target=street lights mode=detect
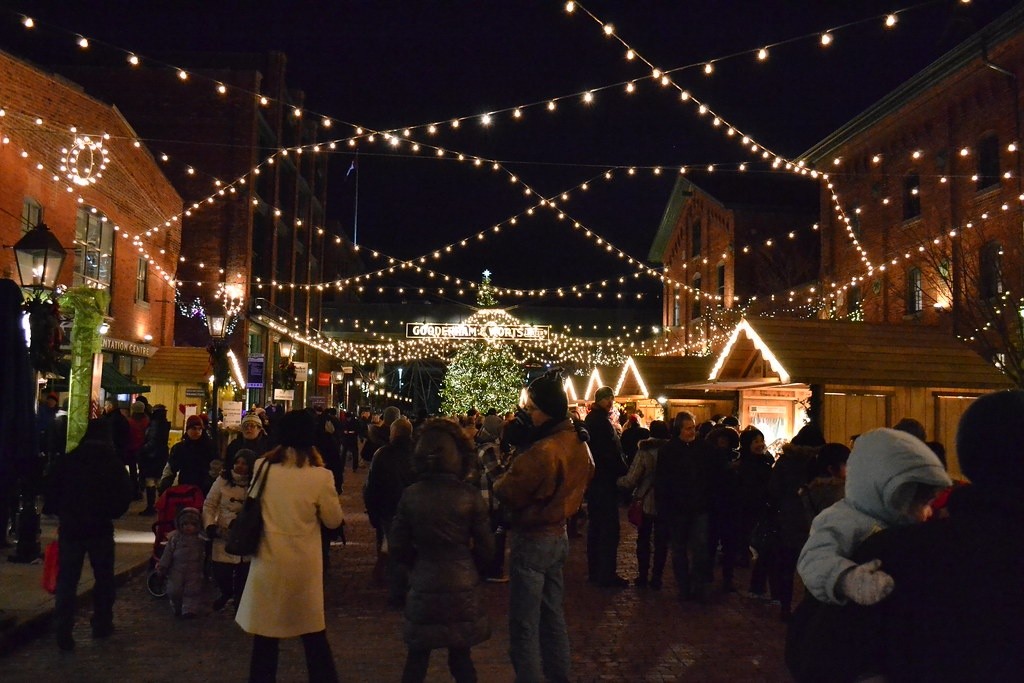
[335,364,345,411]
[279,333,296,413]
[12,224,68,559]
[204,300,228,457]
[368,379,376,411]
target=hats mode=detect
[529,368,566,393]
[815,443,852,477]
[47,391,59,402]
[233,449,257,471]
[179,513,202,530]
[131,402,145,413]
[186,414,210,431]
[629,415,637,423]
[530,379,568,420]
[241,414,262,428]
[956,390,1024,487]
[595,386,615,402]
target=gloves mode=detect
[576,425,592,442]
[839,559,894,606]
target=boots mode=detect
[566,515,583,537]
[137,483,158,517]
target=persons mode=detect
[225,412,270,469]
[774,424,825,491]
[38,391,68,465]
[788,390,1024,683]
[203,449,256,613]
[156,507,206,615]
[796,427,951,612]
[490,369,594,683]
[34,418,131,649]
[157,416,218,495]
[241,405,413,564]
[388,417,495,683]
[453,409,741,591]
[102,394,171,516]
[926,440,972,517]
[231,410,347,683]
[585,387,630,587]
[496,367,589,537]
[795,443,852,563]
[734,426,783,605]
[895,417,927,443]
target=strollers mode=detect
[146,483,211,597]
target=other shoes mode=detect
[634,573,649,587]
[374,551,389,573]
[485,574,509,583]
[587,572,631,587]
[649,567,664,589]
[7,554,38,563]
[92,622,114,636]
[55,617,75,651]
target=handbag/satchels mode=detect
[629,495,645,525]
[225,457,272,556]
